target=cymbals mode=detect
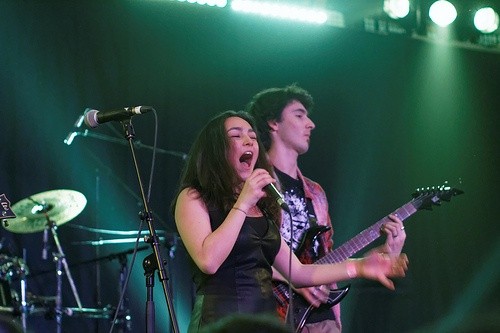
[2,189,87,234]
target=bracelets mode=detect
[347,258,357,277]
[232,207,247,215]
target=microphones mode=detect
[263,184,289,214]
[84,105,154,129]
[63,114,85,145]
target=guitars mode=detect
[273,186,464,332]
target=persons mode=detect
[247,87,406,333]
[171,111,409,333]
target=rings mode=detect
[313,289,319,296]
[315,285,323,290]
[394,235,397,237]
[401,226,404,230]
